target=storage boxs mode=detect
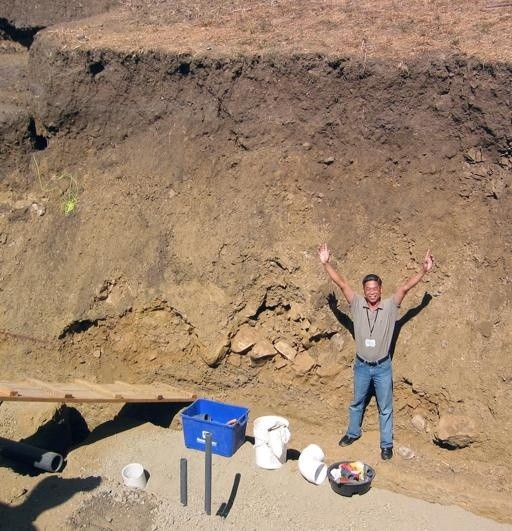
[179,398,250,457]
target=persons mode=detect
[315,242,435,460]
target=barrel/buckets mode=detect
[253,415,290,469]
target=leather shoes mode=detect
[380,446,393,460]
[338,436,360,448]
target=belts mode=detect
[356,354,389,368]
